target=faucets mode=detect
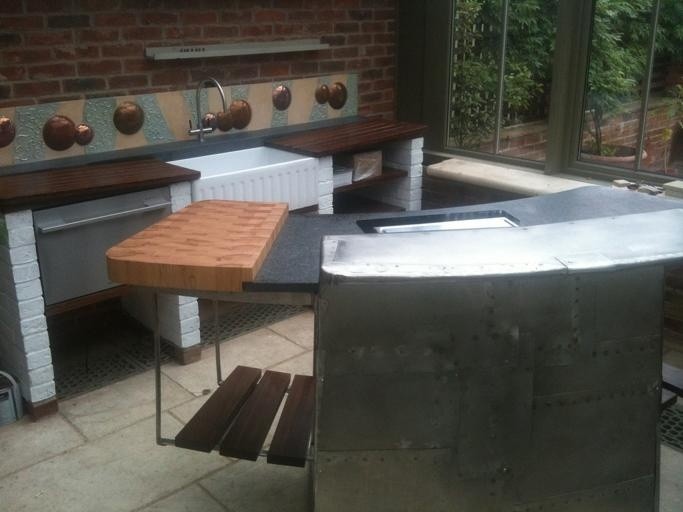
[187,76,227,142]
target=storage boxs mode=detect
[332,149,383,188]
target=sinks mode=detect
[354,210,521,235]
[165,144,318,213]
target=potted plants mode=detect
[577,0,647,170]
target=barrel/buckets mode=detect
[0,372,23,423]
